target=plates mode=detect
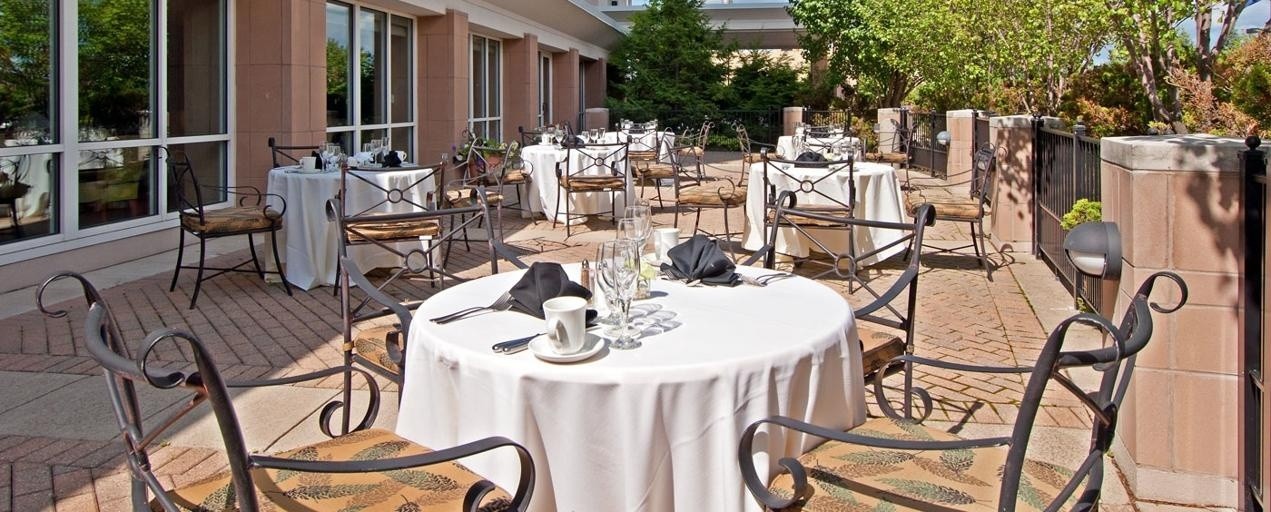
[395,151,406,161]
[297,169,320,174]
[643,252,673,266]
[528,333,604,363]
[539,143,550,146]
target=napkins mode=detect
[659,236,741,287]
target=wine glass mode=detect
[791,122,858,160]
[614,118,659,132]
[319,137,389,173]
[606,303,680,341]
[595,205,652,349]
[547,126,604,148]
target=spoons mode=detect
[741,273,794,287]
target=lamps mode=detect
[1065,220,1122,281]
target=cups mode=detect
[299,156,316,171]
[654,229,679,261]
[542,135,548,144]
[542,296,587,355]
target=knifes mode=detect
[492,323,598,354]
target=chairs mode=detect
[746,126,915,294]
[39,269,536,511]
[663,139,749,244]
[265,138,514,306]
[462,118,714,237]
[903,144,1000,282]
[168,150,294,307]
[739,187,939,427]
[324,187,501,436]
[738,273,1189,510]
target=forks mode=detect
[428,291,514,324]
